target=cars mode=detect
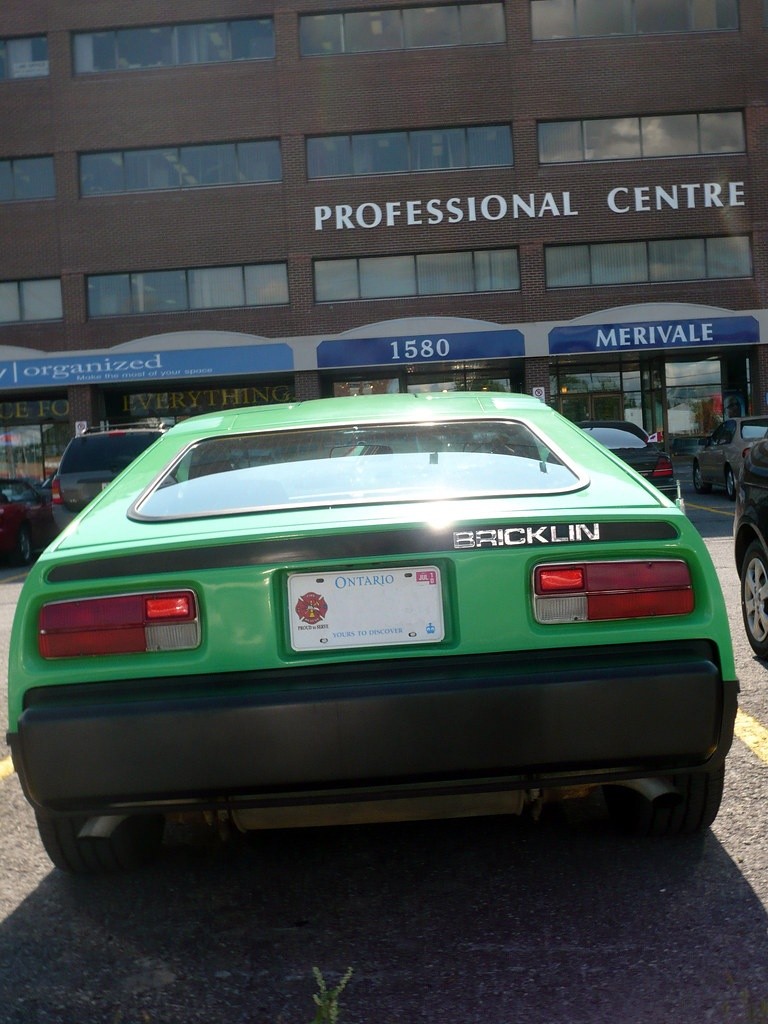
[693,415,768,502]
[733,429,768,663]
[0,479,56,569]
[573,419,677,503]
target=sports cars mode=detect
[6,392,742,909]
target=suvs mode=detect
[50,421,171,533]
